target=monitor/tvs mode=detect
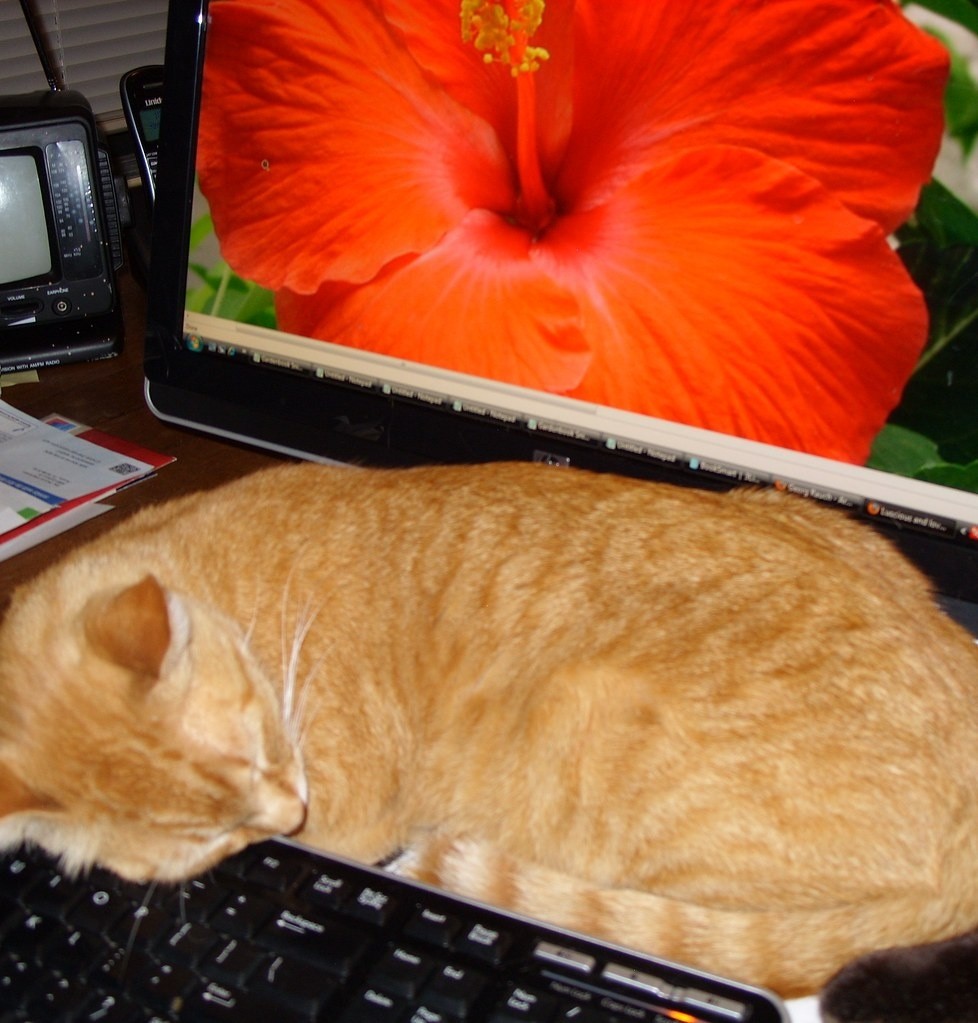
[140,0,978,641]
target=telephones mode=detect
[120,63,164,280]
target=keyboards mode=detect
[1,838,789,1023]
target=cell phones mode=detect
[117,65,166,202]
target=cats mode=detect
[0,462,978,1003]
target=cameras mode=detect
[1,89,122,374]
